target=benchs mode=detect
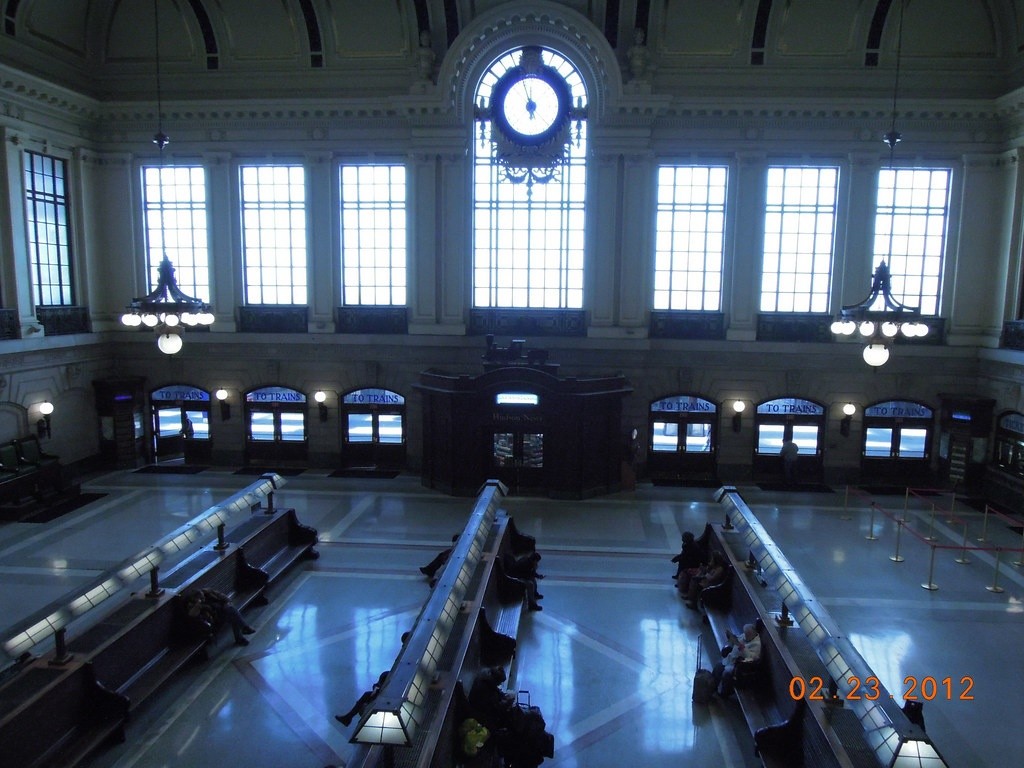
[695,522,854,768]
[0,508,321,768]
[340,483,536,768]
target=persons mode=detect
[682,550,728,608]
[671,532,706,588]
[420,535,459,578]
[781,437,799,487]
[503,552,542,611]
[193,587,256,646]
[711,624,762,699]
[517,553,544,599]
[335,671,390,727]
[468,665,506,708]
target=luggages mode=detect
[692,633,716,704]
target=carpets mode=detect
[1007,527,1024,535]
[859,485,915,496]
[18,493,110,523]
[327,470,401,479]
[651,478,724,488]
[955,497,997,514]
[232,467,307,477]
[132,466,211,474]
[756,483,835,493]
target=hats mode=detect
[491,665,507,682]
[682,532,694,541]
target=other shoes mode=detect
[241,627,256,635]
[237,637,250,645]
[675,584,679,588]
[528,602,542,612]
[672,574,678,578]
[419,566,435,578]
[685,601,698,610]
[535,592,543,599]
[335,715,352,727]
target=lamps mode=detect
[348,479,509,747]
[831,0,929,366]
[122,0,215,354]
[314,389,327,423]
[0,473,287,659]
[37,400,55,439]
[715,486,950,768]
[215,386,231,421]
[840,401,856,438]
[732,399,746,433]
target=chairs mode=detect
[0,435,63,503]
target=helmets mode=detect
[722,645,732,658]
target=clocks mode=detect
[489,50,573,168]
